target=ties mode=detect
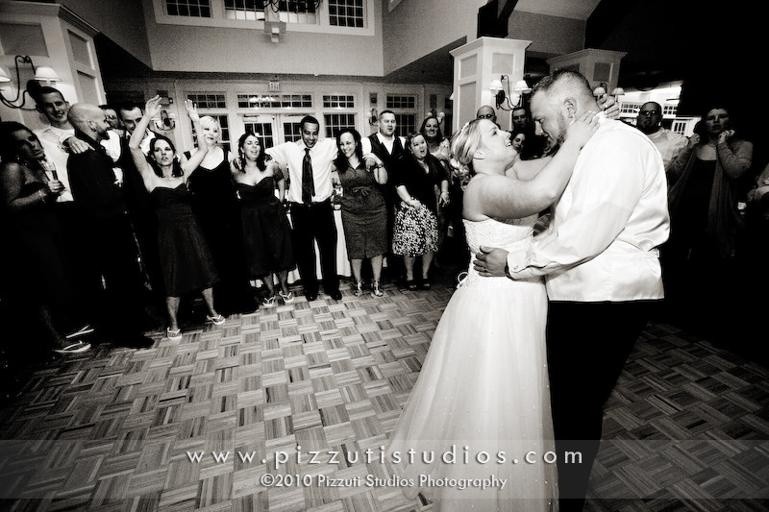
[302,147,315,207]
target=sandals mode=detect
[369,278,382,296]
[352,279,363,297]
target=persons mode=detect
[0,67,769,512]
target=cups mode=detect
[41,161,61,194]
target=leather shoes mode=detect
[406,277,431,291]
[305,290,341,302]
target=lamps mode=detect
[592,82,624,101]
[491,74,531,110]
[152,112,175,131]
[0,54,58,111]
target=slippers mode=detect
[262,294,279,308]
[207,312,226,327]
[167,325,182,340]
[278,289,295,303]
[66,325,94,340]
[52,340,91,354]
